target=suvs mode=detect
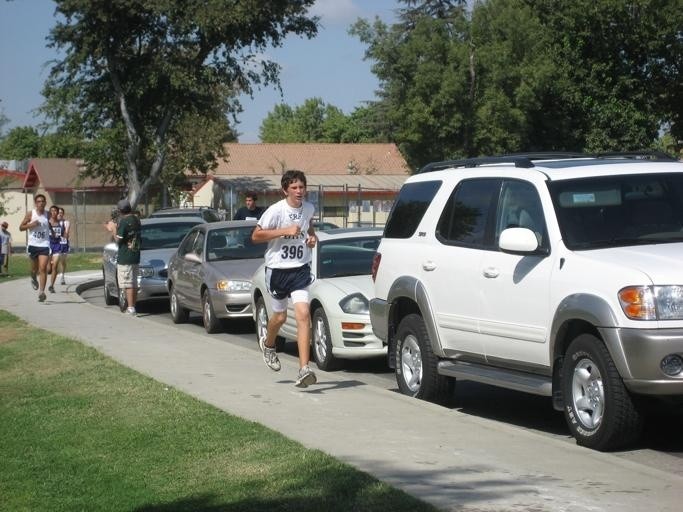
[369,150,682,452]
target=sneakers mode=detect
[31,276,65,303]
[259,336,282,372]
[122,309,137,318]
[294,366,317,389]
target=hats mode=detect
[117,199,131,210]
[0,221,9,226]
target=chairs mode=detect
[624,190,673,237]
[210,235,226,252]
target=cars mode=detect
[168,218,269,335]
[148,204,220,224]
[249,225,392,370]
[101,217,209,312]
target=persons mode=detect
[250,170,317,388]
[233,193,265,221]
[20,194,52,302]
[104,199,141,317]
[0,221,11,274]
[47,205,64,293]
[56,208,71,285]
